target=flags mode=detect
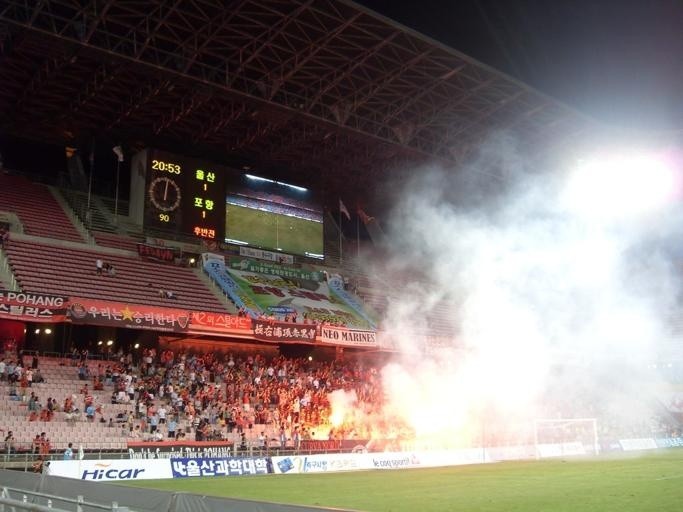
[356,204,373,225]
[339,199,351,220]
[112,145,124,162]
[63,131,81,164]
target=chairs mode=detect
[0,351,163,449]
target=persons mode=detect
[106,262,115,277]
[37,432,48,458]
[166,288,177,299]
[61,346,385,451]
[95,256,103,276]
[238,307,346,328]
[158,286,164,299]
[0,339,60,422]
[31,457,42,473]
[45,437,51,453]
[63,441,73,461]
[4,430,16,458]
[30,434,40,455]
